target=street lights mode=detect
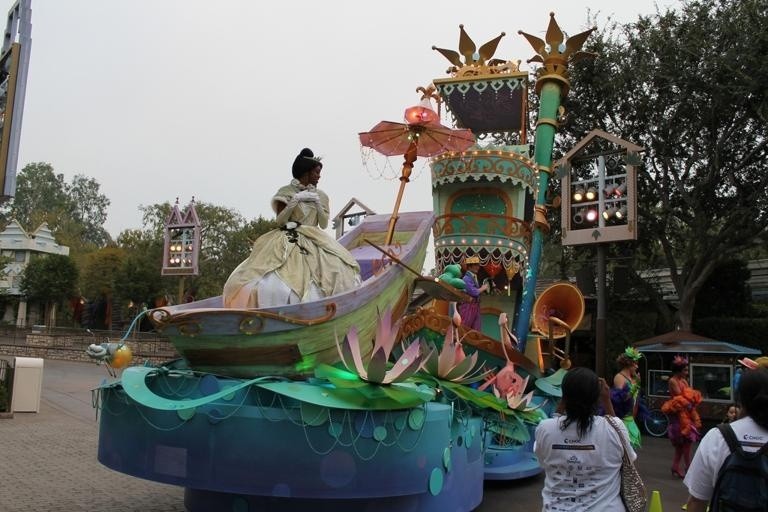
[160,197,202,308]
[552,129,647,380]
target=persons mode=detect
[661,356,768,512]
[223,148,362,309]
[533,366,638,512]
[612,350,643,451]
[460,256,487,332]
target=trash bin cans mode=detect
[11,356,44,414]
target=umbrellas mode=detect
[357,118,478,246]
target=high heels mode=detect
[671,467,685,479]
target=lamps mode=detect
[573,177,628,224]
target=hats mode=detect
[464,257,480,264]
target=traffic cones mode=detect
[648,490,664,512]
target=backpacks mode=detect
[709,424,768,512]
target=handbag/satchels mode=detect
[605,414,647,512]
